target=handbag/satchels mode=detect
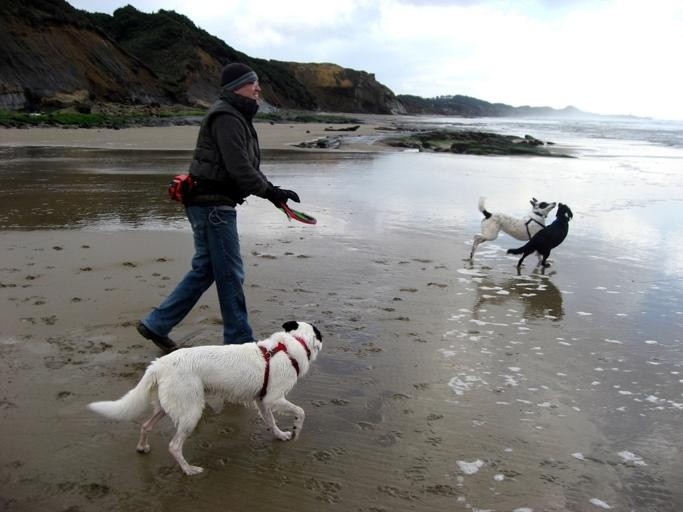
[169,173,197,203]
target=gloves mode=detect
[264,186,289,208]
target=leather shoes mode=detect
[137,322,178,353]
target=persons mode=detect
[136,61,301,353]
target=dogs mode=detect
[85,320,325,477]
[506,202,573,268]
[470,196,557,259]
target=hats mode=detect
[220,62,258,95]
